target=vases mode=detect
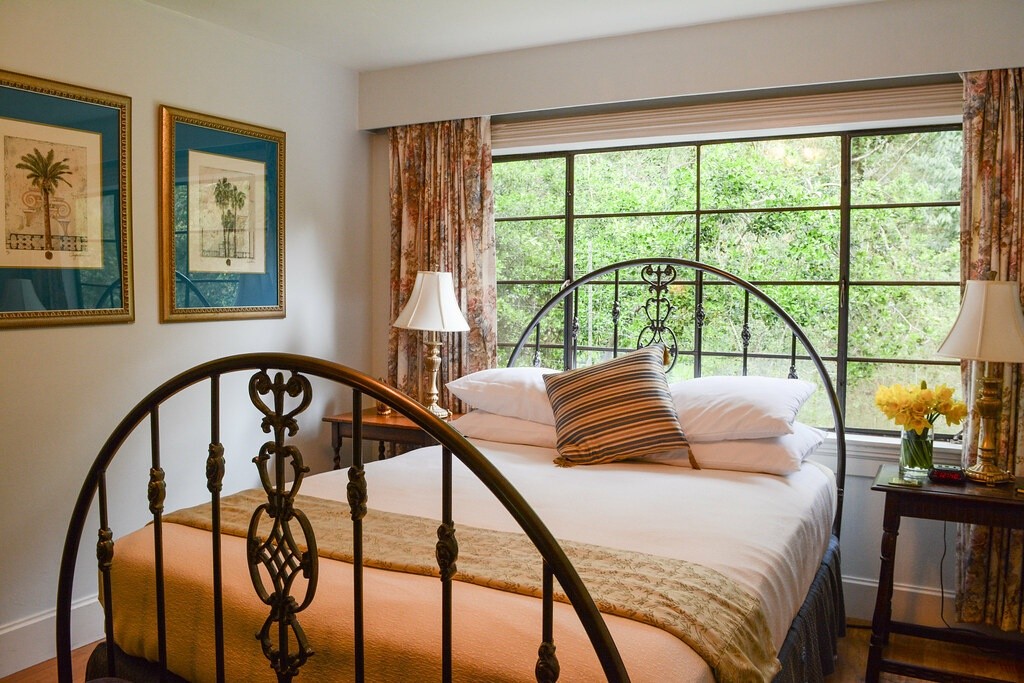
[900,427,936,478]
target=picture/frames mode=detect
[1,69,137,327]
[156,104,285,325]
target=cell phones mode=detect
[887,475,923,487]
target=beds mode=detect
[51,256,849,683]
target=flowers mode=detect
[876,378,970,469]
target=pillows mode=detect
[539,342,690,470]
[668,374,820,444]
[448,409,558,449]
[445,366,562,427]
[690,418,830,477]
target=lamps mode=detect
[932,279,1024,485]
[392,269,472,418]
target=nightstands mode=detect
[861,458,1024,683]
[322,401,441,475]
